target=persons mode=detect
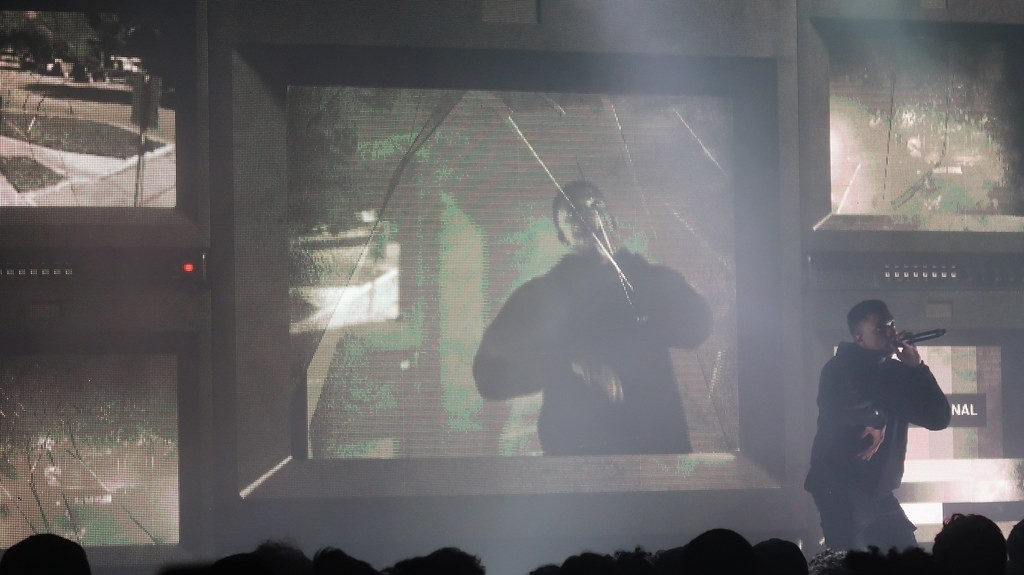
[470,178,717,457]
[803,298,952,552]
[2,513,1024,574]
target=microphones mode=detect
[894,329,946,347]
